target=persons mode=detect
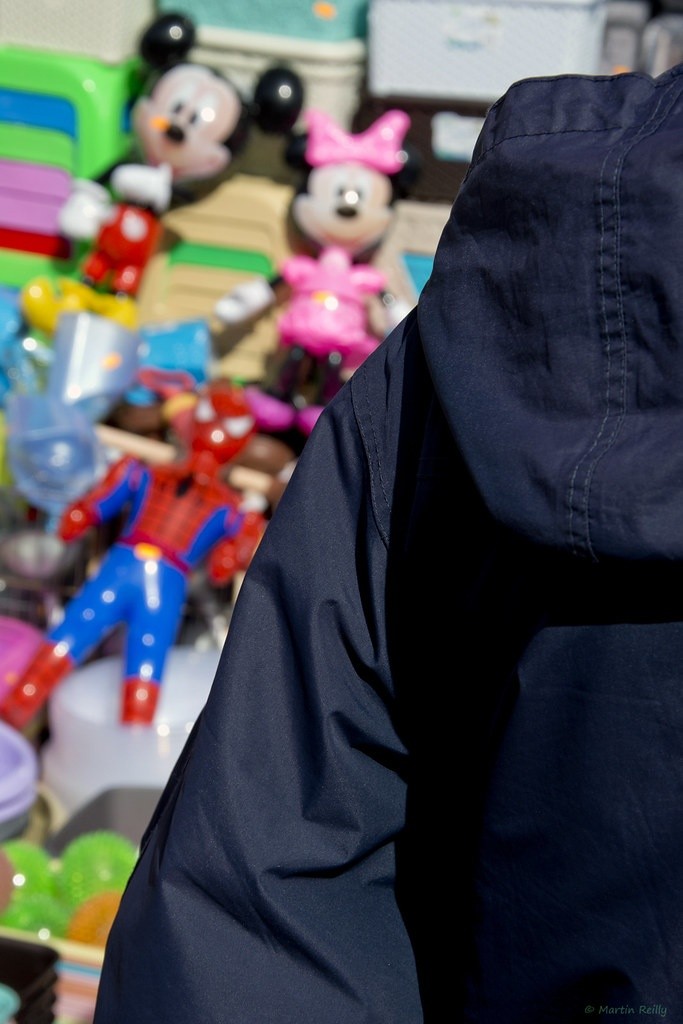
[83,0,682,1024]
[2,381,269,741]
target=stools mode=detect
[1,39,451,403]
